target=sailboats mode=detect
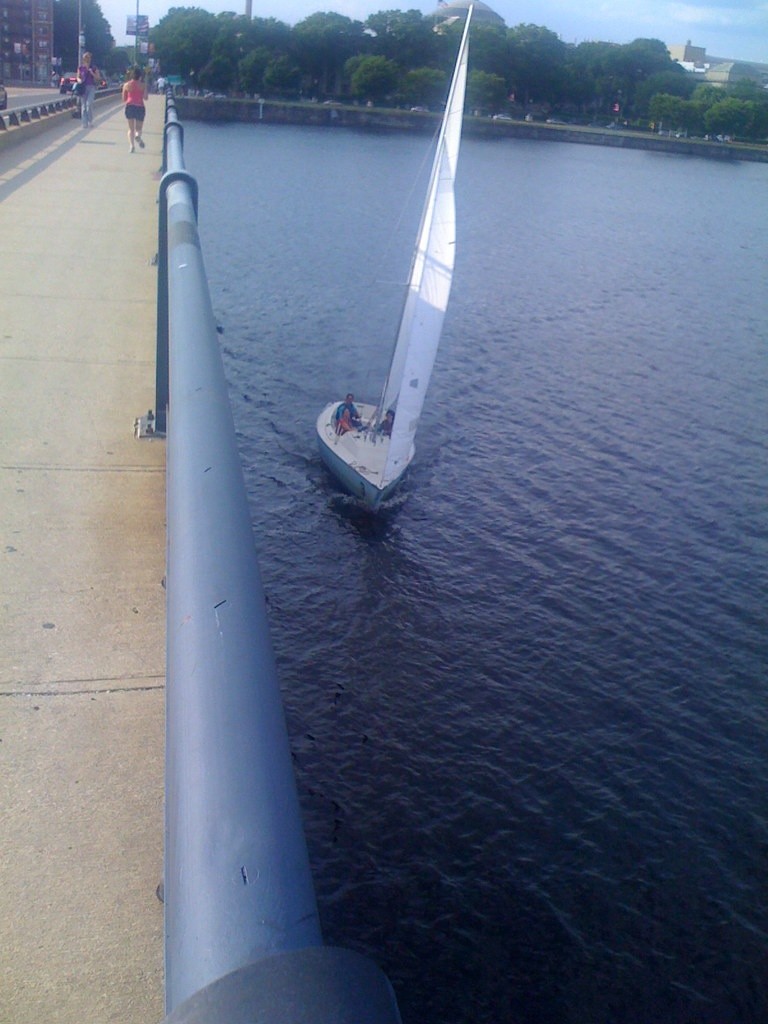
[314,3,475,508]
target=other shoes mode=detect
[135,136,144,148]
[130,147,135,153]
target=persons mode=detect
[157,75,166,95]
[52,69,60,88]
[76,51,100,129]
[377,410,395,436]
[122,68,148,153]
[334,394,368,436]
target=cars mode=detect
[411,106,430,112]
[59,72,78,94]
[95,68,110,90]
[323,99,343,108]
[205,92,228,100]
[493,114,512,121]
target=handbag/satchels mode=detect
[72,81,85,95]
